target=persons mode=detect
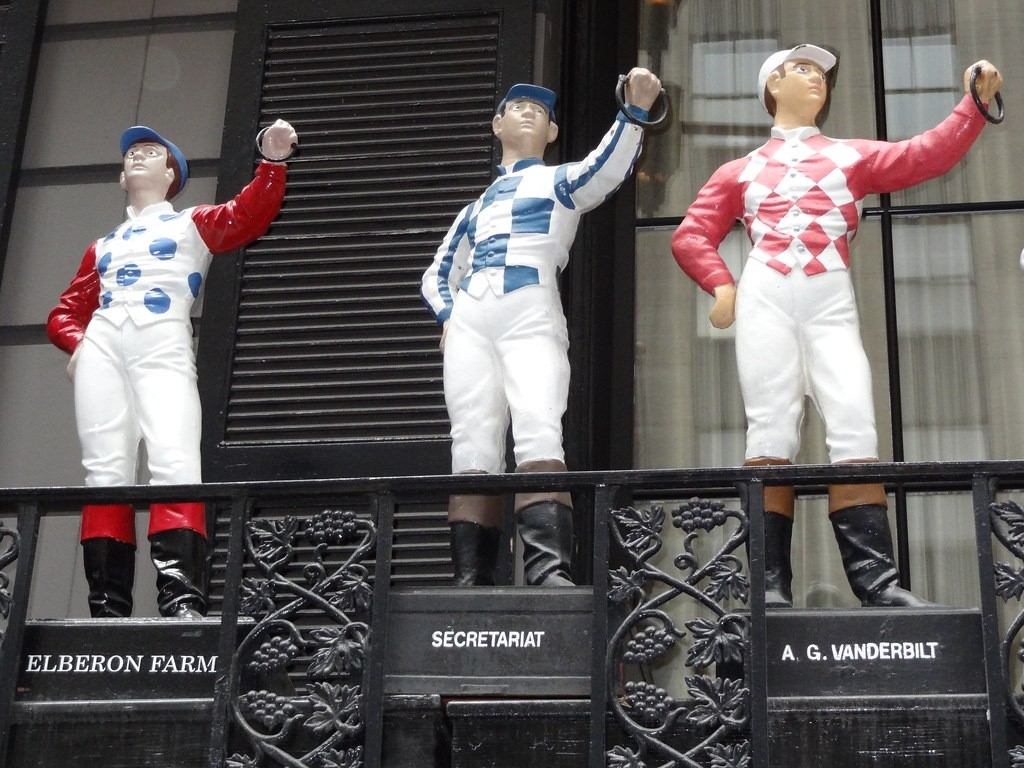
[670,40,1006,611]
[44,117,301,618]
[421,66,677,590]
